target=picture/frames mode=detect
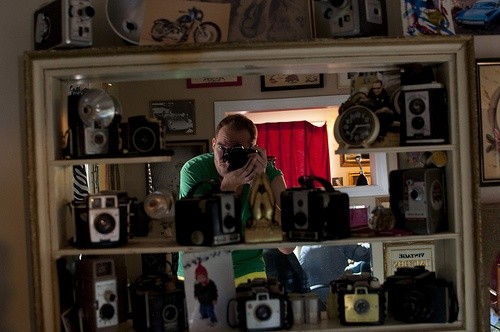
[476,58,500,187]
[349,172,372,186]
[340,154,371,167]
[383,242,434,281]
[149,98,198,136]
[145,139,209,197]
[186,75,242,89]
[260,73,325,92]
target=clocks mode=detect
[334,92,380,149]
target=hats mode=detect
[195,265,207,275]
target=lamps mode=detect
[355,156,369,185]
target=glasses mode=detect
[372,87,381,90]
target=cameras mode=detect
[33,0,458,332]
[223,144,259,171]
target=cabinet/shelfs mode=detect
[21,32,491,332]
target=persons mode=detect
[178,114,373,311]
[193,264,219,325]
[359,79,396,138]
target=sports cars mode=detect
[455,0,500,27]
